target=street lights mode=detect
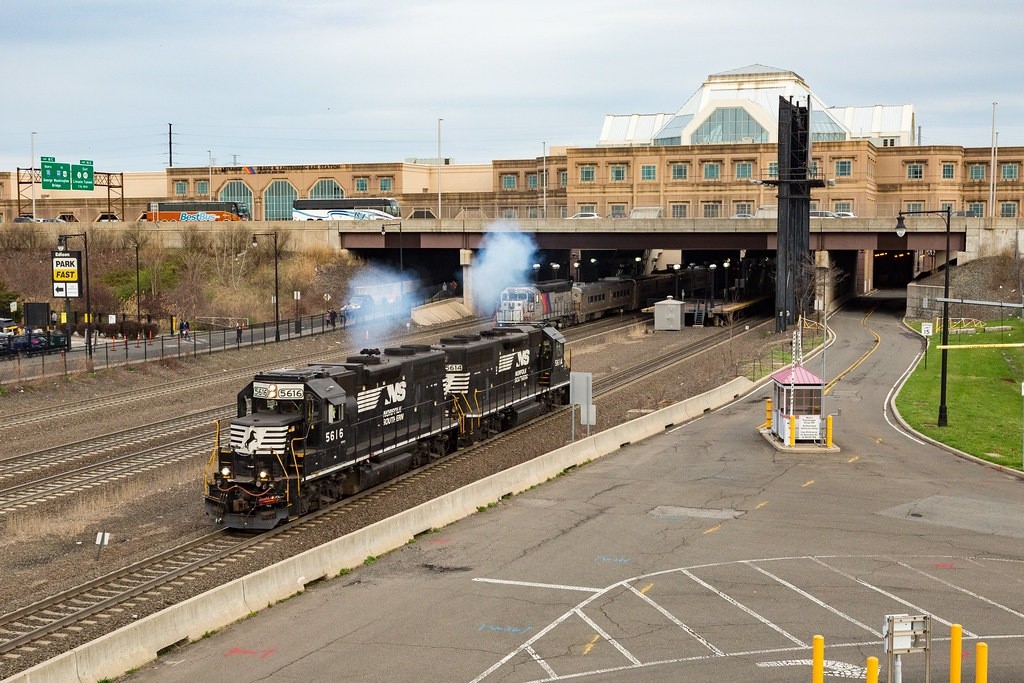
[207,150,211,201]
[382,222,404,309]
[253,232,280,342]
[989,101,1000,217]
[895,207,951,427]
[673,262,730,308]
[32,131,37,223]
[439,118,444,219]
[542,141,546,218]
[57,232,92,359]
[533,257,641,282]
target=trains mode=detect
[204,324,572,530]
[495,266,740,329]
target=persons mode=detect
[442,281,448,297]
[236,327,242,343]
[51,310,58,325]
[179,319,190,340]
[326,308,337,324]
[450,280,457,297]
[544,345,552,369]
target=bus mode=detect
[292,198,401,221]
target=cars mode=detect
[14,217,64,223]
[147,201,250,222]
[0,318,48,356]
[346,296,377,318]
[733,211,856,218]
[564,213,602,219]
